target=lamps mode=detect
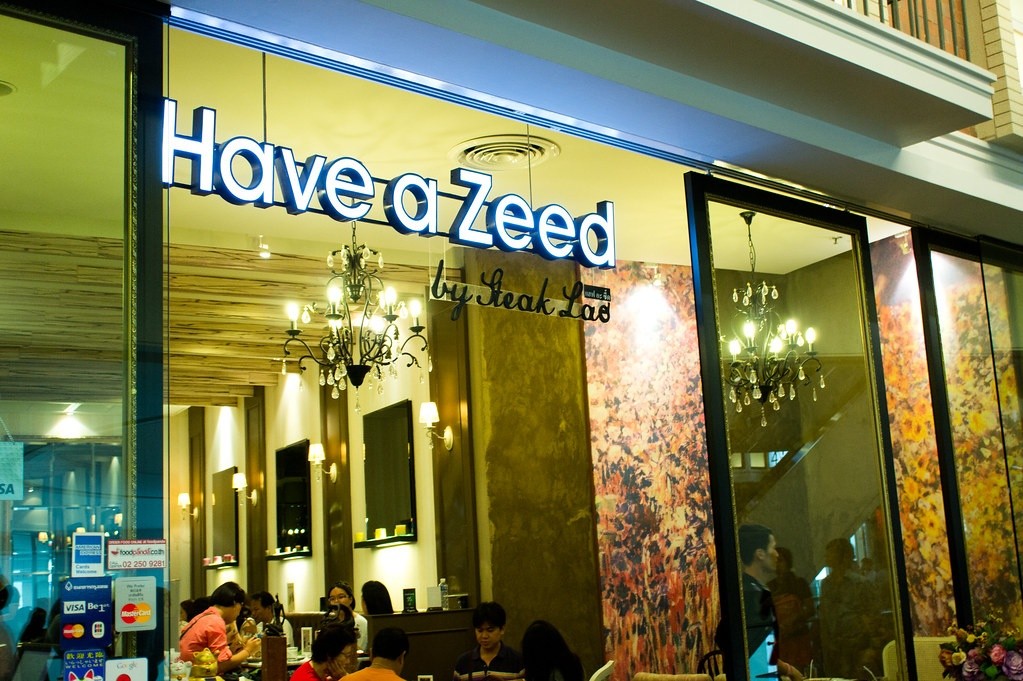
[177,493,199,520]
[419,402,454,451]
[307,443,338,484]
[722,211,826,427]
[282,194,434,416]
[231,473,257,507]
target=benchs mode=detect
[279,611,335,652]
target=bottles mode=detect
[438,578,449,610]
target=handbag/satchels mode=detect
[773,593,804,635]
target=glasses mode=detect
[341,651,352,659]
[328,594,349,602]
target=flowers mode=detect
[936,609,1023,681]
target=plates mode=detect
[246,658,262,663]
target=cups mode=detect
[287,647,298,660]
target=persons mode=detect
[178,581,587,681]
[738,522,890,681]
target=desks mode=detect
[238,651,370,681]
